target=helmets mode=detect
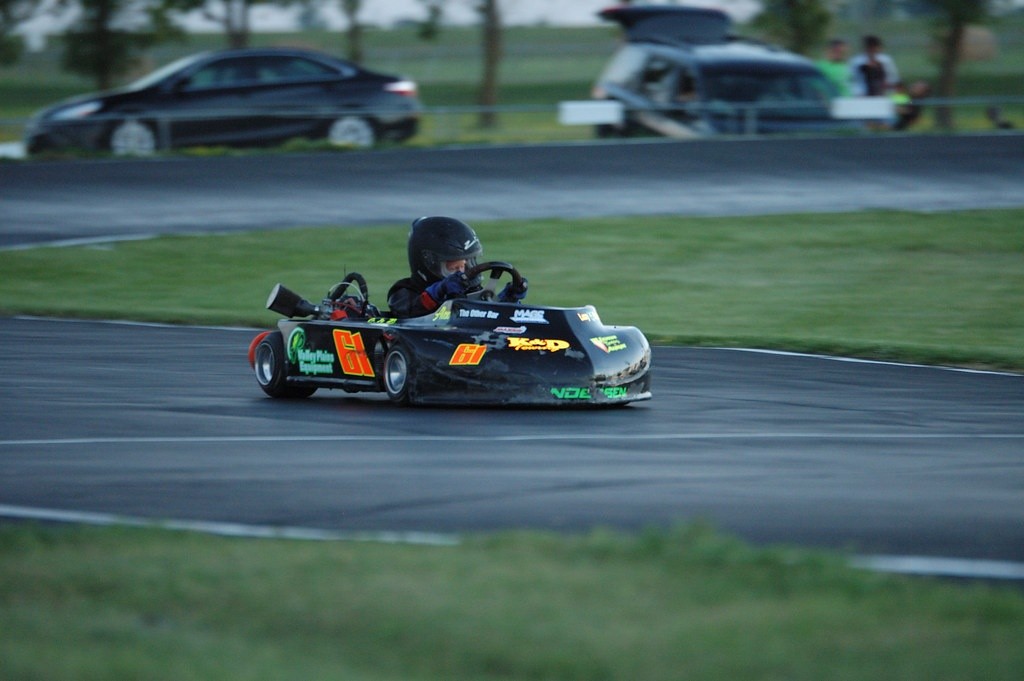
[408,217,483,297]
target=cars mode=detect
[26,46,420,156]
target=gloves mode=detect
[497,277,528,304]
[425,271,482,305]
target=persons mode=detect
[820,38,852,96]
[849,35,900,97]
[387,215,529,318]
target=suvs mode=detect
[591,5,897,139]
